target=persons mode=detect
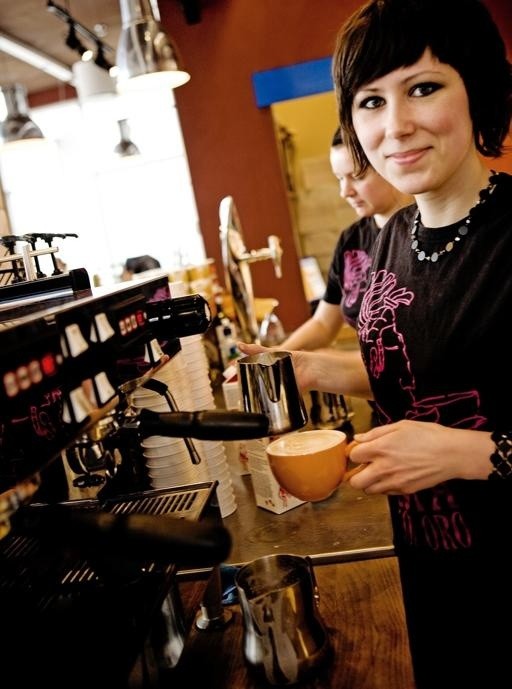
[235,1,512,689]
[272,126,415,352]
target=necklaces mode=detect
[410,170,499,263]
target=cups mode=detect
[266,428,370,504]
[127,332,239,520]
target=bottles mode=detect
[221,329,247,368]
[236,553,336,689]
[308,389,357,430]
[235,350,309,436]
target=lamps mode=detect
[65,19,116,78]
[0,84,44,143]
[116,0,192,91]
[114,118,138,156]
[71,60,117,111]
